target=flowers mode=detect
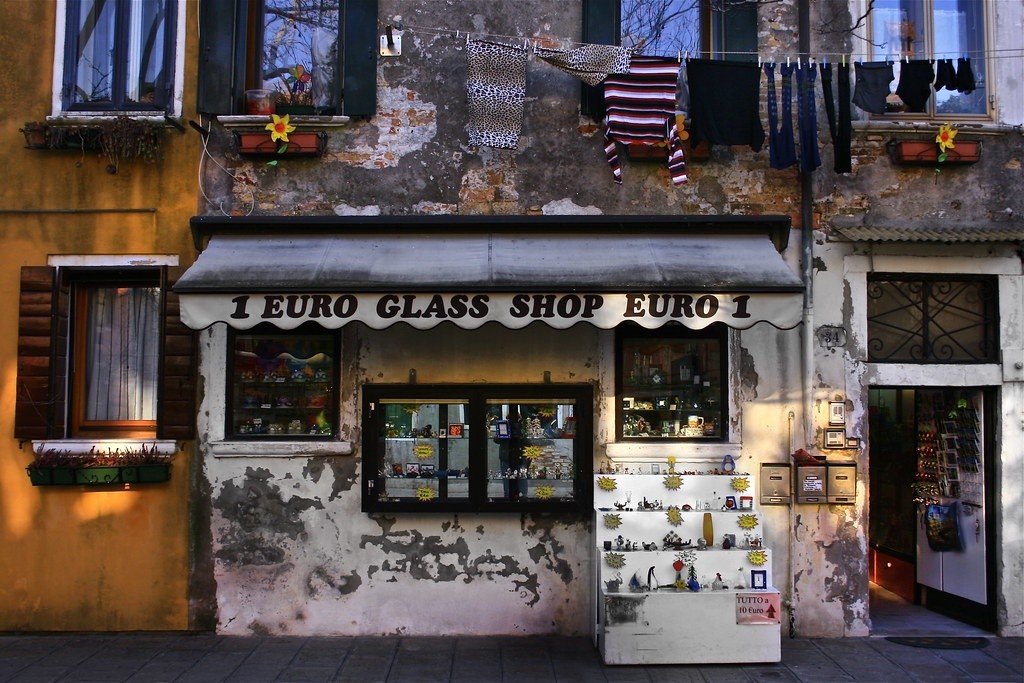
[264,113,297,176]
[287,63,311,94]
[934,122,958,185]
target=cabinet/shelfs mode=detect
[593,474,782,664]
[385,436,574,480]
[624,383,705,412]
[235,380,330,414]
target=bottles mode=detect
[629,353,653,385]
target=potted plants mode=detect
[19,121,101,147]
[27,441,172,487]
[273,84,315,115]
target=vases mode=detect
[239,132,319,152]
[896,141,978,161]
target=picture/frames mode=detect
[449,422,464,438]
[496,420,511,438]
[421,464,435,470]
[941,421,960,481]
[406,463,420,476]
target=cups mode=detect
[703,513,713,546]
[688,415,704,429]
[245,89,275,114]
